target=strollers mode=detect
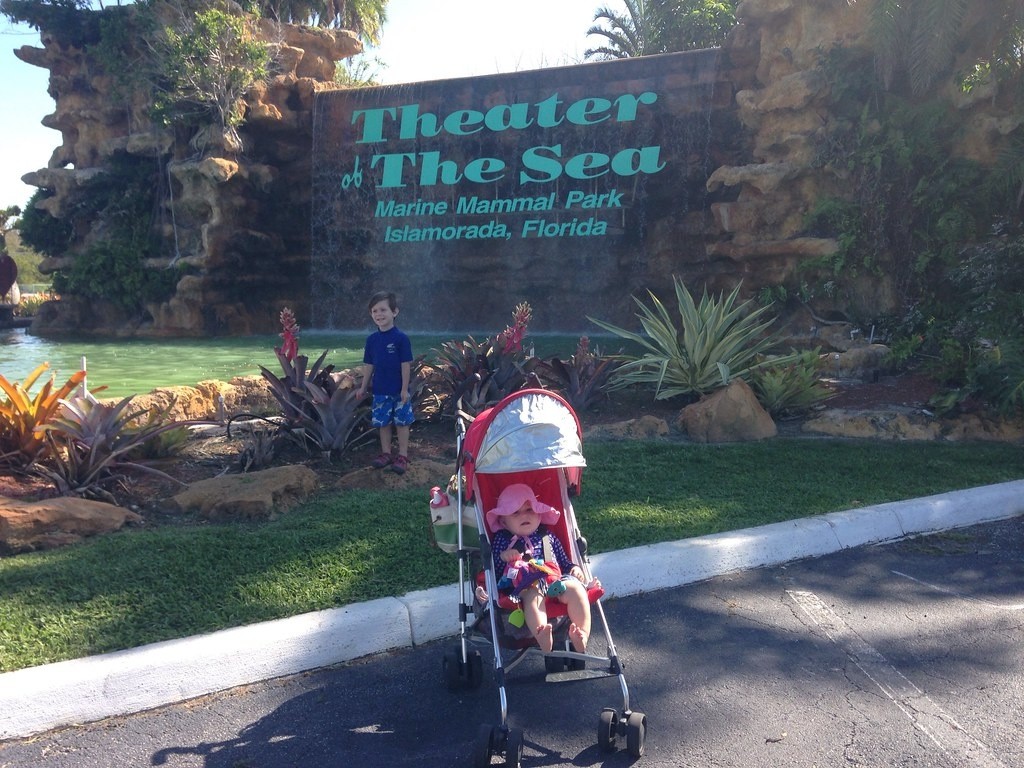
[427,387,651,768]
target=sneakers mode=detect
[392,454,411,473]
[372,453,392,467]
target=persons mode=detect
[487,484,590,653]
[356,292,416,473]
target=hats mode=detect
[486,484,561,533]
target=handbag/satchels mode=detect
[430,441,481,553]
